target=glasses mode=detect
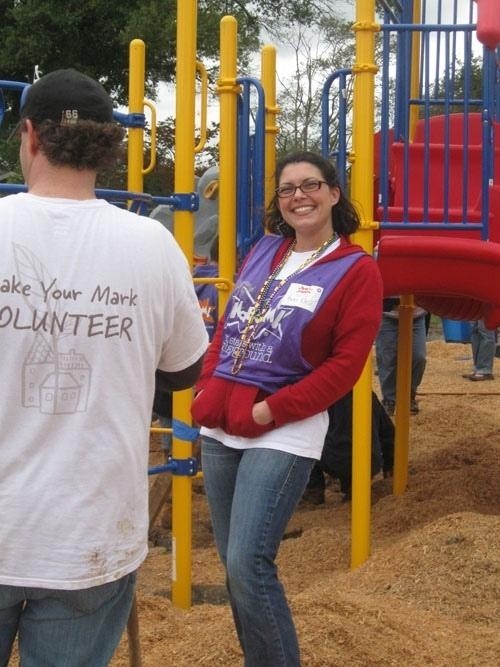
[274,179,330,198]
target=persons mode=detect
[373,295,428,417]
[190,151,384,667]
[461,318,495,381]
[0,70,210,666]
[297,387,395,505]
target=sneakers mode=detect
[383,397,419,416]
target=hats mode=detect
[6,66,116,145]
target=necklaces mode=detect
[231,228,340,376]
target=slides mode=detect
[365,110,500,332]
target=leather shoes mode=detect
[462,370,494,382]
